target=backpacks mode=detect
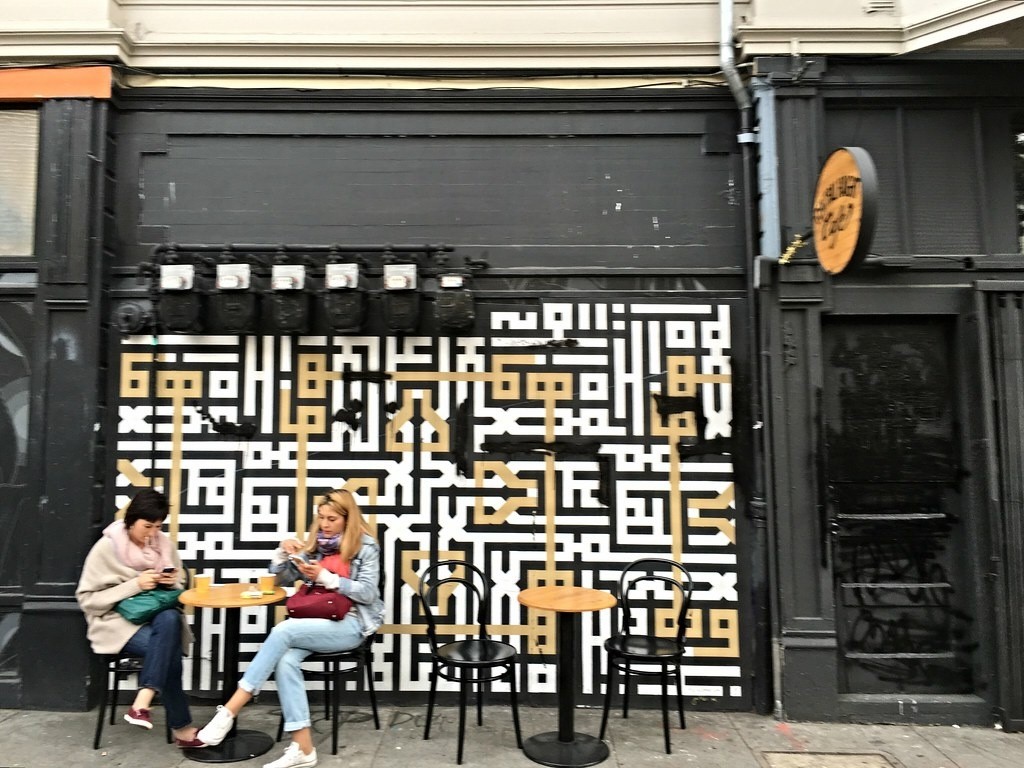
[285,553,354,619]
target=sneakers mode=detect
[123,706,153,729]
[197,705,234,745]
[176,729,208,748]
[263,742,318,768]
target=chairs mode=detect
[92,563,190,751]
[598,558,694,755]
[277,571,386,755]
[418,559,524,765]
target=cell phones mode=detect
[157,568,175,590]
[293,557,305,566]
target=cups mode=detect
[194,574,209,598]
[257,573,277,593]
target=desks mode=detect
[517,586,618,768]
[178,583,286,763]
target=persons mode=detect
[77,490,208,747]
[198,490,384,767]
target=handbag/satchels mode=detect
[115,587,185,625]
